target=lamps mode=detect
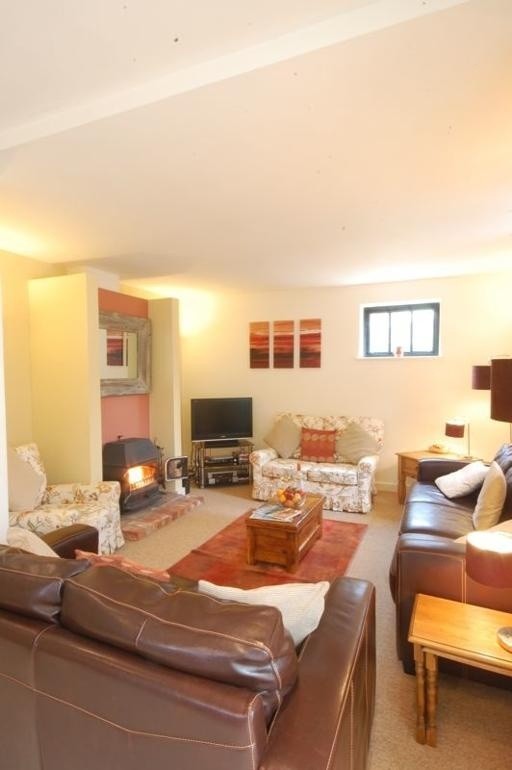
[444,415,476,458]
[470,365,489,391]
[489,358,512,445]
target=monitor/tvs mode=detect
[191,397,252,448]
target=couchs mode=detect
[7,439,126,557]
[388,444,512,651]
[0,522,378,770]
[248,411,385,513]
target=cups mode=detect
[278,476,306,508]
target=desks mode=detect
[395,448,464,507]
[406,592,511,749]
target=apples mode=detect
[284,488,305,507]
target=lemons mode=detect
[277,488,286,504]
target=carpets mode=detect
[164,509,368,587]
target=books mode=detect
[249,502,301,522]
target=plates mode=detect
[497,625,512,654]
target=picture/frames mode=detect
[99,308,152,398]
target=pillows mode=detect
[472,462,507,531]
[300,425,339,464]
[262,413,301,459]
[195,576,330,654]
[333,421,382,464]
[433,459,487,499]
[74,548,171,581]
[7,526,61,557]
